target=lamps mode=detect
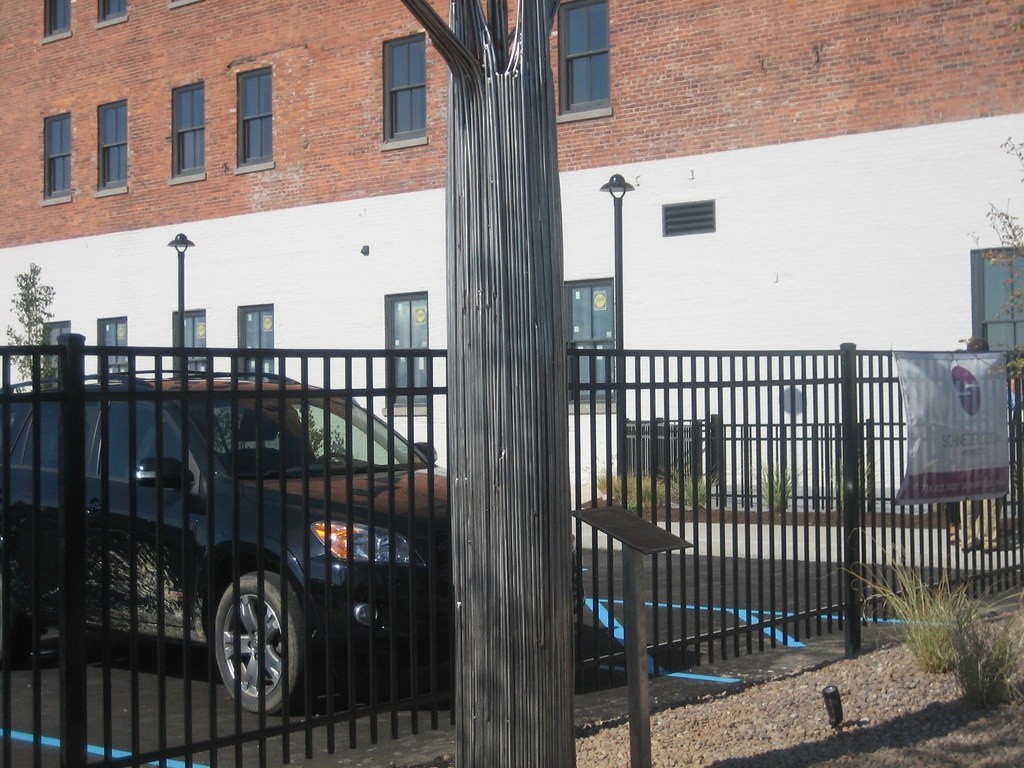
[821,686,844,727]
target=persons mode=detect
[945,349,967,545]
[961,338,1016,553]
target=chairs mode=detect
[140,422,188,490]
[237,403,298,473]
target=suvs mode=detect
[0,367,588,718]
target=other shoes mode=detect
[949,530,958,545]
[983,541,1013,553]
[961,540,982,552]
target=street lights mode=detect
[166,233,196,377]
[599,171,635,485]
